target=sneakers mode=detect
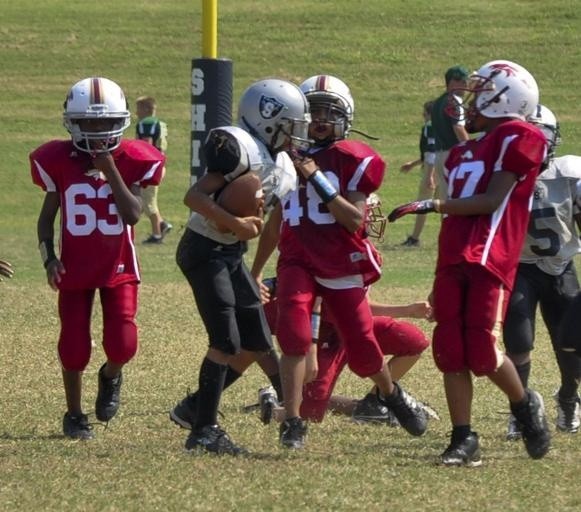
[553,384,580,434]
[93,361,125,421]
[507,386,551,461]
[392,236,423,251]
[254,383,311,450]
[168,391,248,457]
[60,410,94,441]
[350,376,430,438]
[141,220,174,246]
[433,431,484,470]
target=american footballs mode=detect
[214,173,266,234]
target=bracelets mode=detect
[308,169,339,203]
[36,240,56,267]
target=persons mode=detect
[28,78,165,439]
[134,96,173,246]
[0,260,14,282]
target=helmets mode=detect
[523,103,560,157]
[235,79,313,155]
[62,76,132,155]
[296,74,357,144]
[443,60,542,127]
